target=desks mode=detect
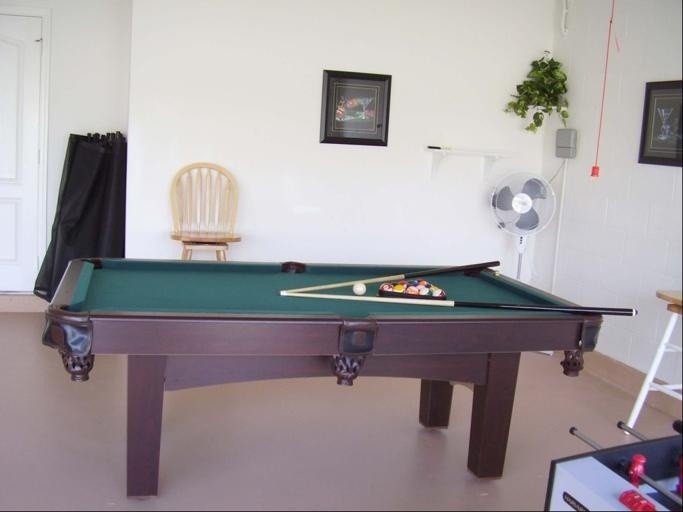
[41,255,604,497]
[622,291,683,437]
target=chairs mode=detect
[170,162,241,263]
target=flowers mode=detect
[490,170,556,281]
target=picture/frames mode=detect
[319,69,392,147]
[637,79,683,166]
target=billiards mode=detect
[353,282,366,295]
[380,281,445,297]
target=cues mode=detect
[279,262,500,292]
[280,292,638,316]
